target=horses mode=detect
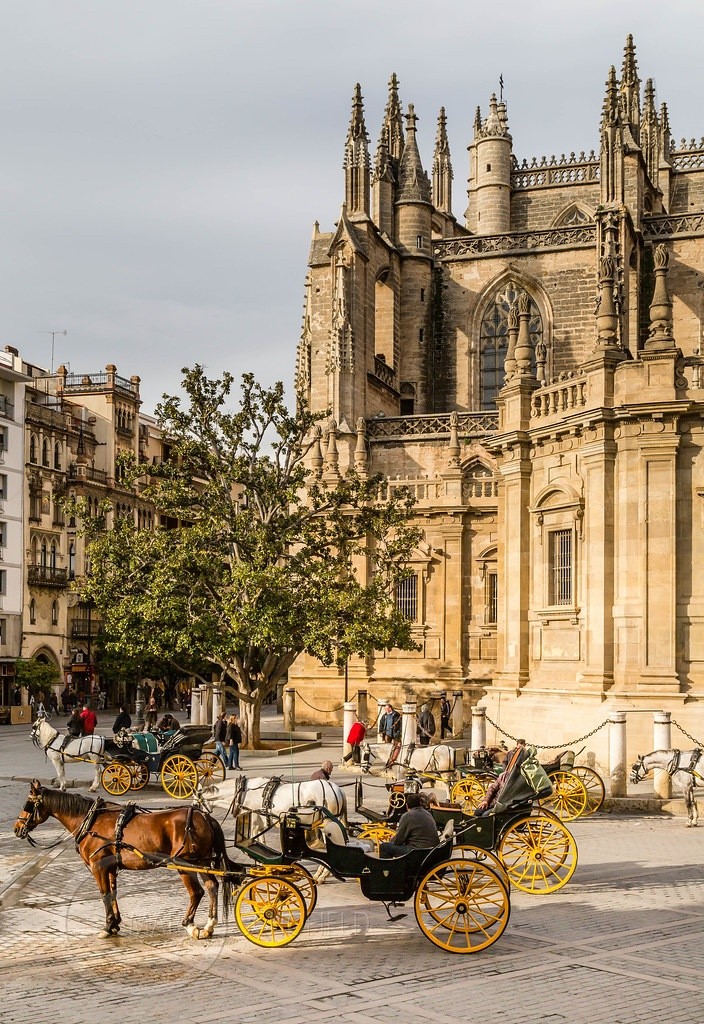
[28,715,106,794]
[11,777,239,941]
[357,740,457,804]
[627,747,704,829]
[189,773,348,888]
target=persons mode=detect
[225,714,244,771]
[66,710,82,737]
[502,739,526,770]
[12,685,84,715]
[475,771,507,816]
[80,705,98,737]
[342,721,367,766]
[112,707,131,734]
[211,711,230,767]
[417,704,436,747]
[439,697,453,739]
[378,704,402,744]
[377,792,441,859]
[141,681,192,744]
[309,760,333,781]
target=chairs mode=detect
[474,745,499,757]
[391,779,404,793]
[280,806,322,825]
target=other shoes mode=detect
[354,762,361,766]
[229,766,235,770]
[235,766,242,770]
[341,757,346,767]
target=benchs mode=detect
[463,800,533,850]
[150,748,203,771]
[371,819,454,902]
[541,750,575,782]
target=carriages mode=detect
[188,741,607,896]
[13,778,514,956]
[27,715,227,800]
